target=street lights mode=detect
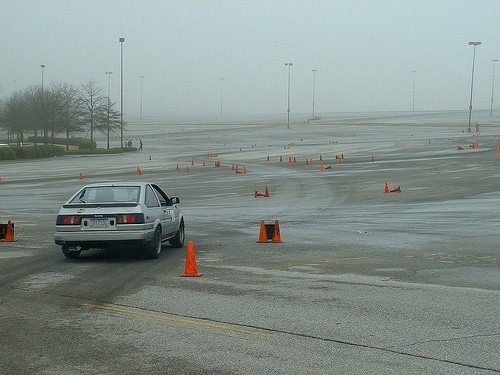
[218,77,225,118]
[40,38,145,150]
[490,60,498,115]
[411,70,417,112]
[468,42,482,132]
[285,63,318,129]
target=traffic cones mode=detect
[224,139,338,151]
[0,219,17,242]
[191,160,220,167]
[80,171,83,181]
[180,241,203,277]
[137,166,142,175]
[186,166,190,173]
[371,133,500,161]
[0,176,2,182]
[305,153,344,170]
[266,155,296,163]
[176,163,179,169]
[254,186,271,197]
[382,182,401,193]
[208,152,217,157]
[257,220,284,243]
[232,163,247,175]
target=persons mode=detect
[125,139,132,146]
[138,139,143,150]
[475,122,479,132]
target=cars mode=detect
[0,144,10,147]
[53,182,184,259]
